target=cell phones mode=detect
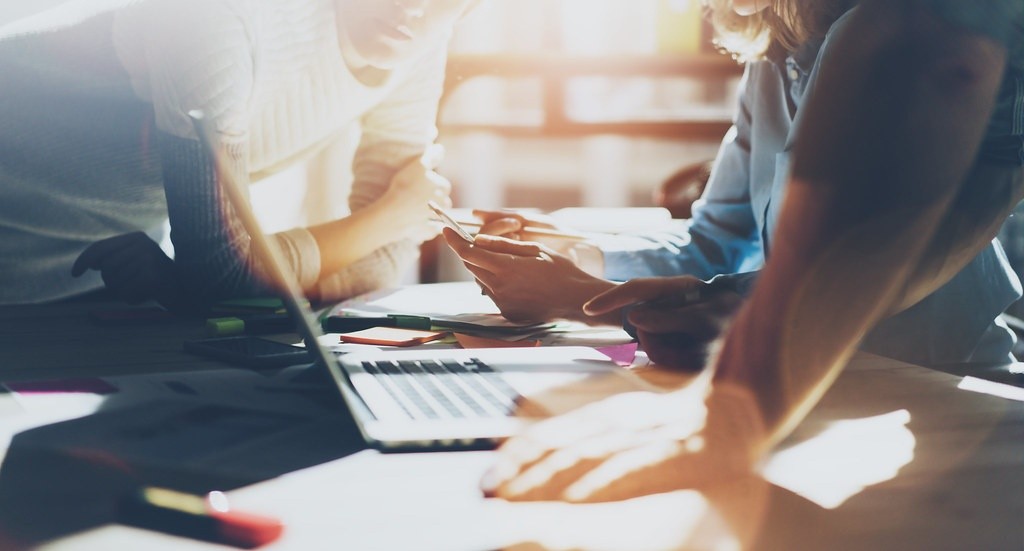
[427,201,476,244]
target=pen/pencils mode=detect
[425,216,597,244]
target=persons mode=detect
[1,1,466,305]
[442,0,1022,387]
[478,0,1023,503]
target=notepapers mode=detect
[340,326,446,348]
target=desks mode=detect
[0,279,1024,551]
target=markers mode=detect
[325,313,434,332]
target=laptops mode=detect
[187,109,672,454]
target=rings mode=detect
[480,286,487,295]
[502,255,514,274]
